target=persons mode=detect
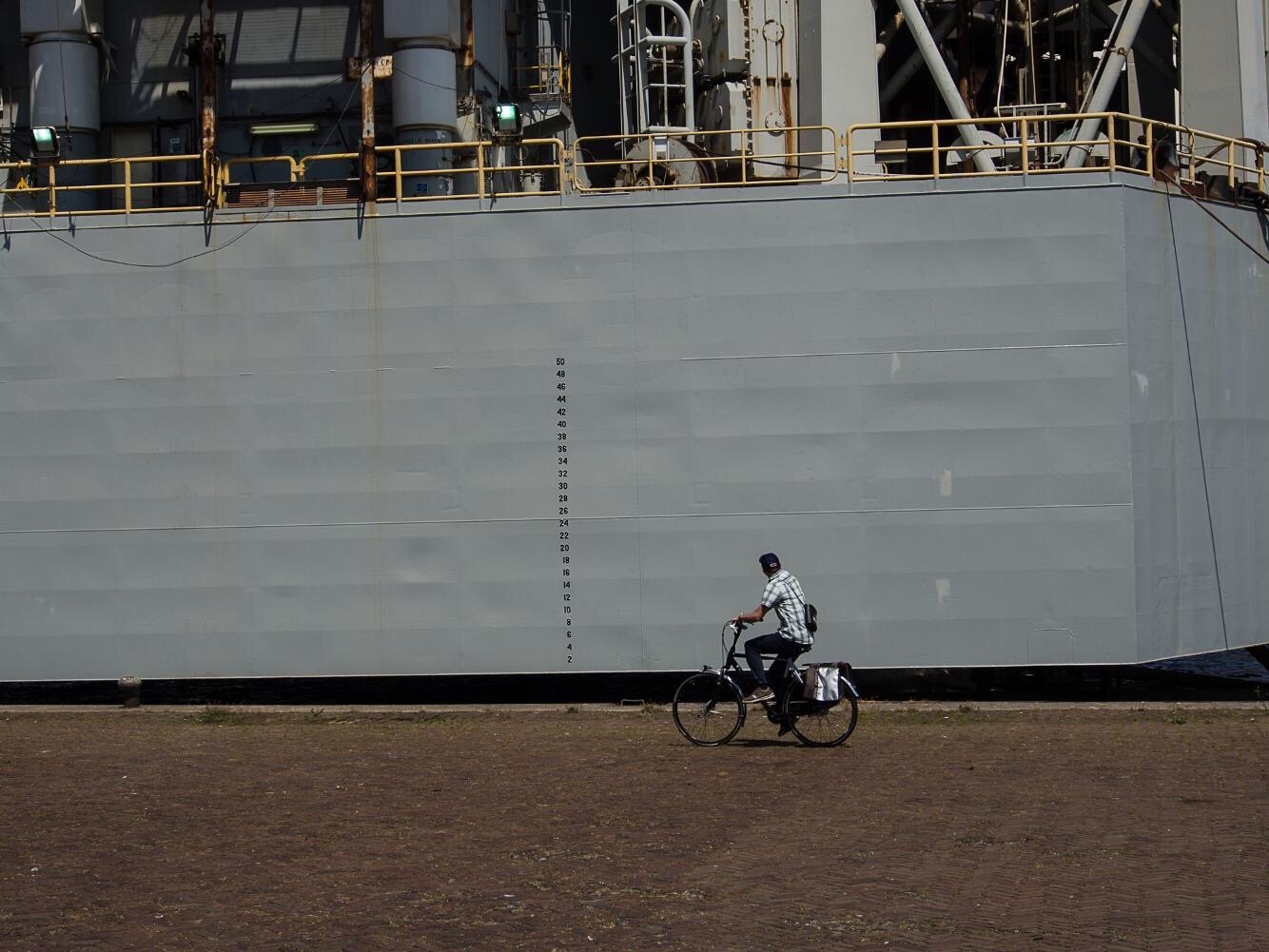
[731,553,815,737]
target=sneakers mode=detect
[741,685,776,703]
[778,715,800,736]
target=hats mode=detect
[759,552,779,568]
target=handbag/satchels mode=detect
[802,660,854,703]
[804,603,818,632]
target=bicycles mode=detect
[672,618,860,747]
